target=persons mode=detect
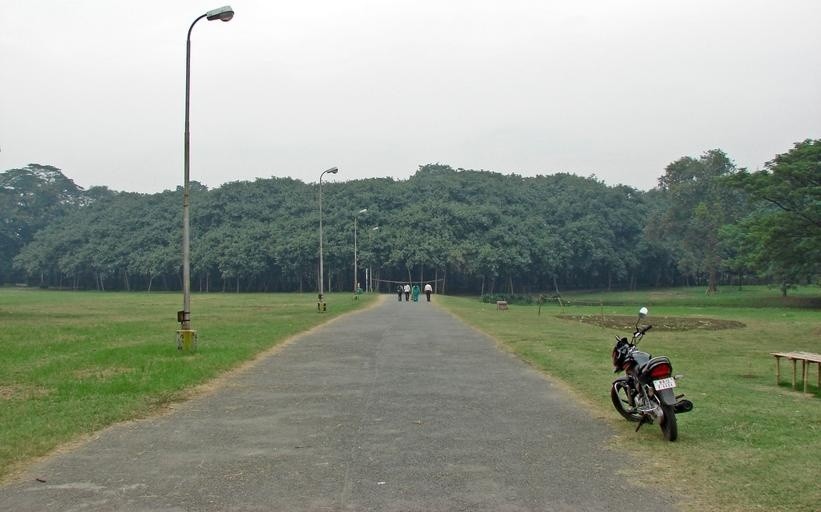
[404,281,412,302]
[396,281,405,301]
[424,281,433,303]
[412,282,422,302]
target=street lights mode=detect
[180,5,235,331]
[365,227,379,293]
[355,209,367,291]
[319,166,338,294]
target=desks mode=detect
[770,349,820,393]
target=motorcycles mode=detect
[609,306,693,442]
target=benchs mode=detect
[497,301,509,312]
